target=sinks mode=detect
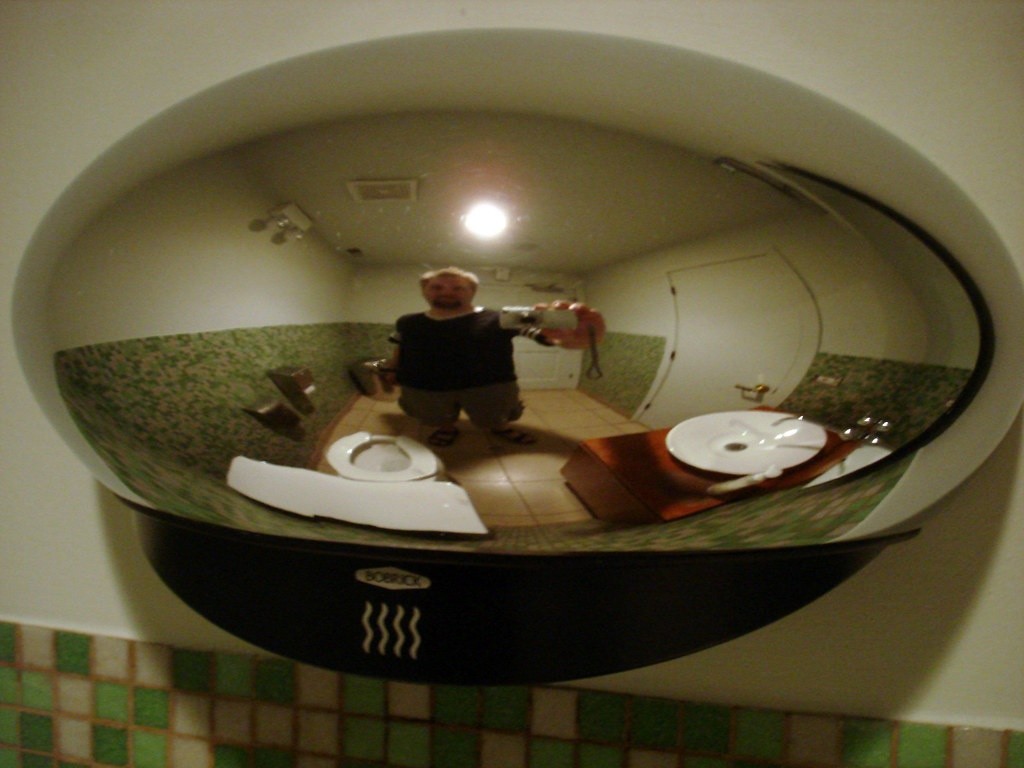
[666,405,829,475]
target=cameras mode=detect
[499,306,577,330]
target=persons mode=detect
[387,266,607,447]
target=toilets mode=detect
[224,430,492,538]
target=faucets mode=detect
[771,409,866,442]
